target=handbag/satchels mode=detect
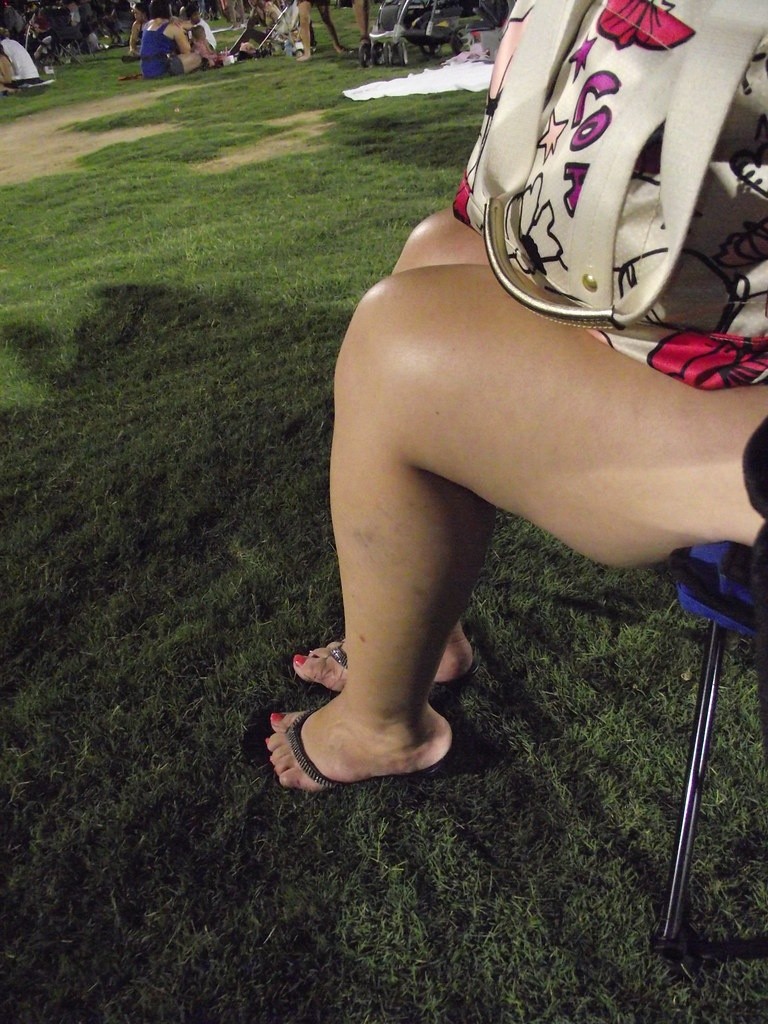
[450,0,768,391]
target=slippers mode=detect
[267,706,460,793]
[302,640,481,694]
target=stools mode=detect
[652,544,768,959]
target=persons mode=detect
[263,204,768,795]
[0,0,385,96]
